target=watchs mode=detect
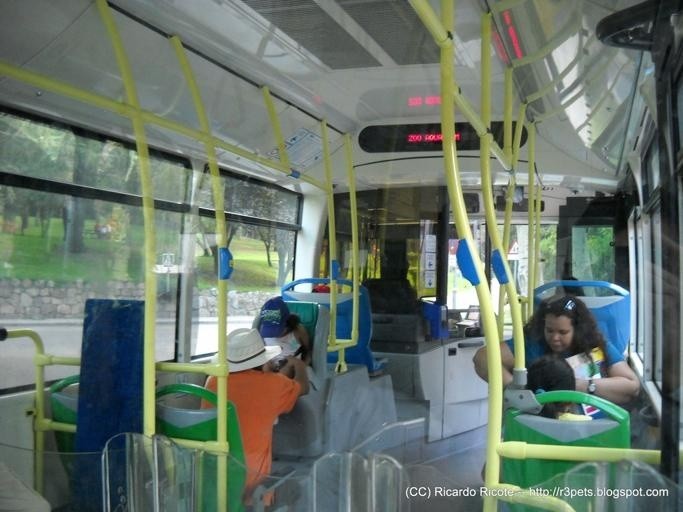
[586,378,596,396]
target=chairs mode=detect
[1,278,683,511]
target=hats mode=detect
[211,328,281,373]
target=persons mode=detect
[478,353,607,485]
[470,294,642,423]
[256,296,311,428]
[198,325,310,512]
[553,275,586,299]
